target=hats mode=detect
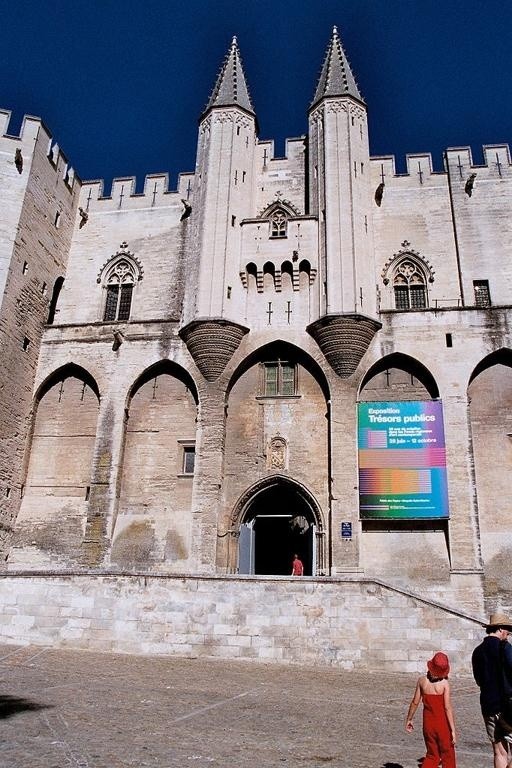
[481,612,512,628]
[425,651,451,678]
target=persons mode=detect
[291,553,304,575]
[404,652,457,767]
[472,612,511,767]
